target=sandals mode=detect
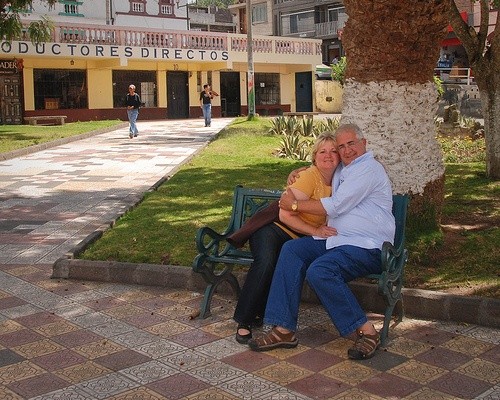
[252,327,298,352]
[347,328,382,359]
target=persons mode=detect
[250,123,396,359]
[232,131,341,344]
[124,84,141,138]
[200,85,213,127]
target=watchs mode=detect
[292,200,298,211]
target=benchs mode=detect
[24,116,67,126]
[192,185,409,347]
[283,112,319,121]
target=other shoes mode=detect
[236,323,253,342]
[134,135,137,137]
[130,135,133,138]
